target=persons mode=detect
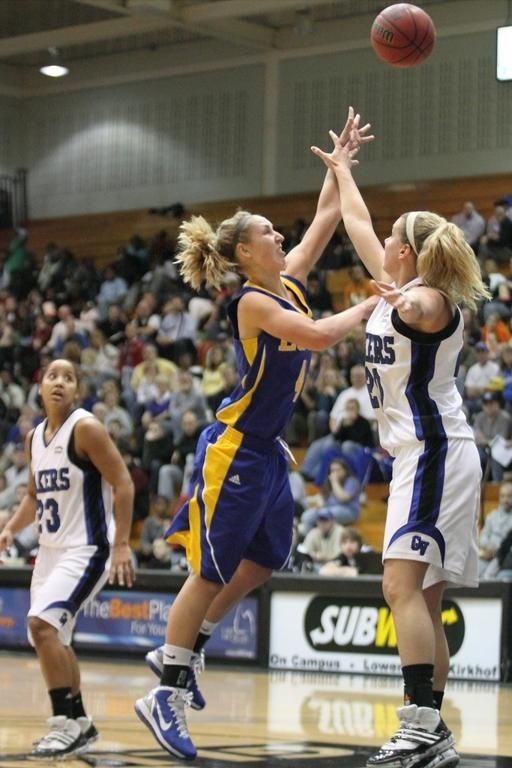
[450,200,490,584]
[0,359,50,567]
[1,359,139,762]
[273,218,309,254]
[309,220,376,576]
[490,195,512,582]
[133,227,239,573]
[309,130,490,767]
[73,359,133,478]
[133,107,377,762]
[0,225,134,359]
[286,382,308,574]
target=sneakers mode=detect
[32,716,100,750]
[145,643,206,710]
[419,748,462,768]
[27,716,88,761]
[367,702,456,767]
[134,686,197,762]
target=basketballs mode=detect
[372,4,435,68]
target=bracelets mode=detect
[113,540,128,547]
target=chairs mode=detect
[313,265,380,317]
[287,439,511,581]
[131,490,189,563]
